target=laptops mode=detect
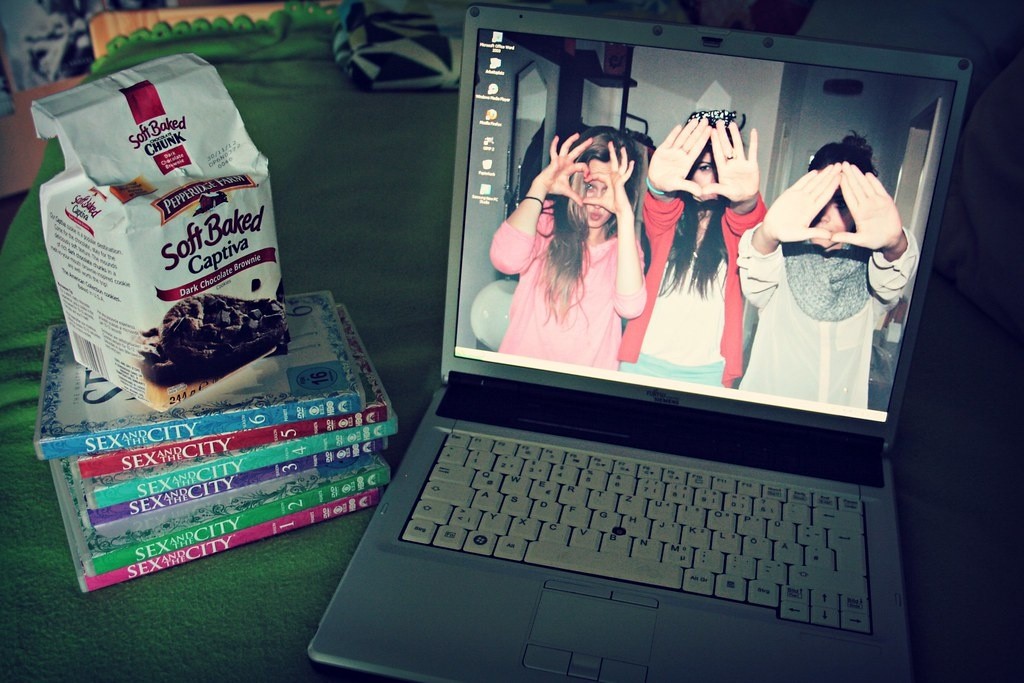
[304,2,972,683]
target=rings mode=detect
[726,157,734,160]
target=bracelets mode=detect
[644,176,678,198]
[519,197,543,213]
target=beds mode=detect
[0,0,1024,683]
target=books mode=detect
[33,290,399,595]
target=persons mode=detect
[489,108,921,410]
